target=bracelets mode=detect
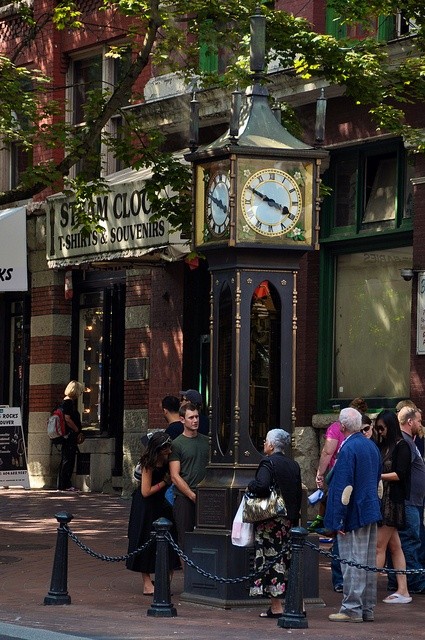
[156,483,160,490]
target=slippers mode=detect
[383,593,413,604]
[302,610,306,616]
[259,607,284,618]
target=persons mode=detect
[169,403,209,570]
[315,397,368,593]
[244,428,307,618]
[386,406,425,593]
[374,409,412,603]
[360,415,378,445]
[179,389,203,414]
[47,380,85,491]
[125,431,182,596]
[162,396,184,440]
[396,399,425,463]
[324,407,384,623]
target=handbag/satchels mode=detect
[308,489,324,505]
[242,457,288,522]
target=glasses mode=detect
[374,425,385,431]
[360,426,370,433]
[395,409,400,413]
[160,436,171,448]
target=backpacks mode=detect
[47,402,67,452]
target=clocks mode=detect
[200,164,232,246]
[236,159,312,243]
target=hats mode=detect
[179,390,202,404]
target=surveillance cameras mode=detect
[402,270,414,284]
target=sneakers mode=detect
[334,583,343,593]
[63,486,76,491]
[328,612,363,623]
